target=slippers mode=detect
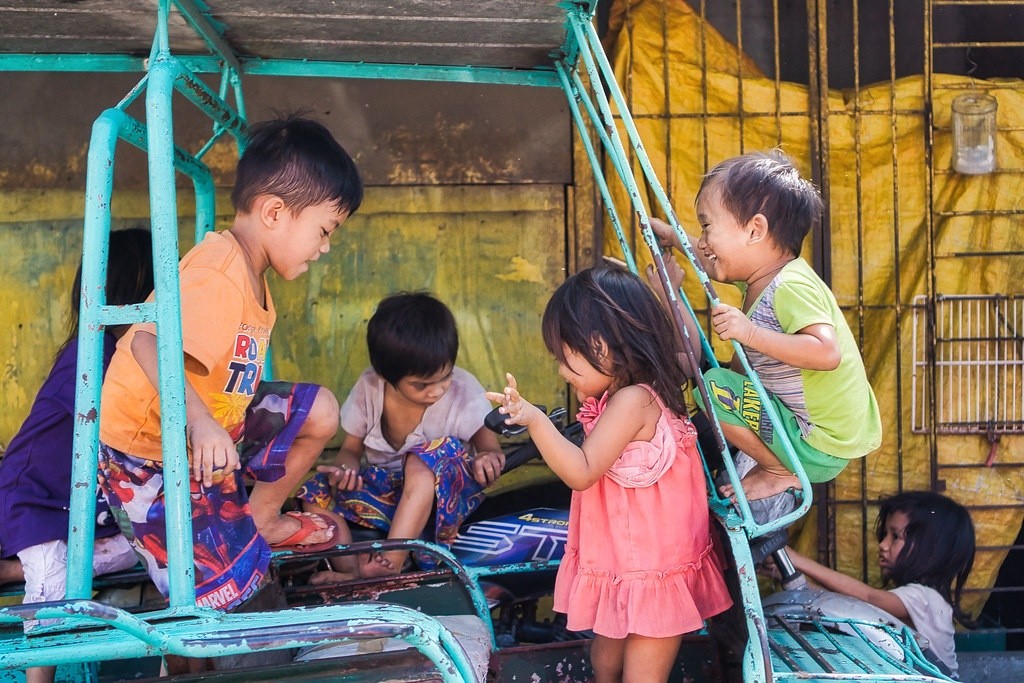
[270,511,340,552]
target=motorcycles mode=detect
[88,400,958,683]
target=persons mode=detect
[98,116,364,616]
[639,150,883,505]
[758,489,977,682]
[294,291,507,586]
[484,244,729,682]
[1,227,182,611]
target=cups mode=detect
[952,92,999,174]
